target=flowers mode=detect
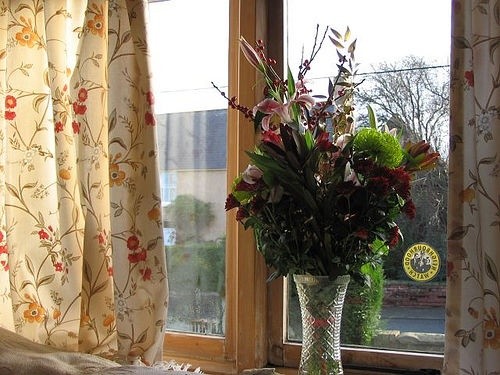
[211,23,442,282]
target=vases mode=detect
[292,274,352,375]
